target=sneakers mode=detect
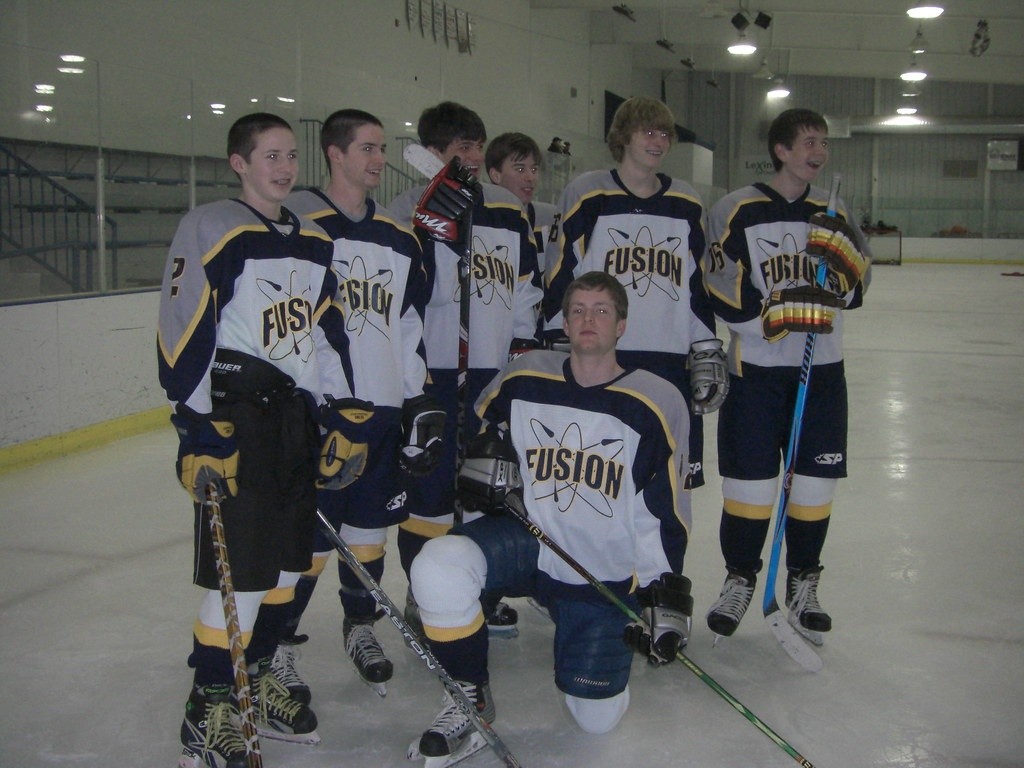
[786,567,831,647]
[271,645,312,705]
[702,556,764,649]
[179,672,249,768]
[407,678,496,768]
[478,603,519,640]
[245,659,322,746]
[342,610,393,697]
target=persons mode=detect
[410,271,695,768]
[706,108,873,651]
[476,131,552,255]
[384,103,544,655]
[270,106,422,697]
[152,114,375,768]
[543,93,729,667]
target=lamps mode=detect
[910,26,930,55]
[899,81,924,95]
[612,0,722,89]
[750,47,775,80]
[727,0,757,54]
[767,55,790,98]
[899,56,927,81]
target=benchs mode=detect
[1,139,240,303]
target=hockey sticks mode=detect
[761,171,844,676]
[206,480,263,768]
[402,140,475,527]
[310,505,520,768]
[494,487,814,768]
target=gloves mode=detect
[412,154,481,242]
[686,338,729,416]
[807,210,865,297]
[167,408,239,506]
[399,394,448,477]
[620,571,692,667]
[760,286,848,343]
[315,396,374,491]
[458,437,527,517]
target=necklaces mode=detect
[618,170,642,212]
